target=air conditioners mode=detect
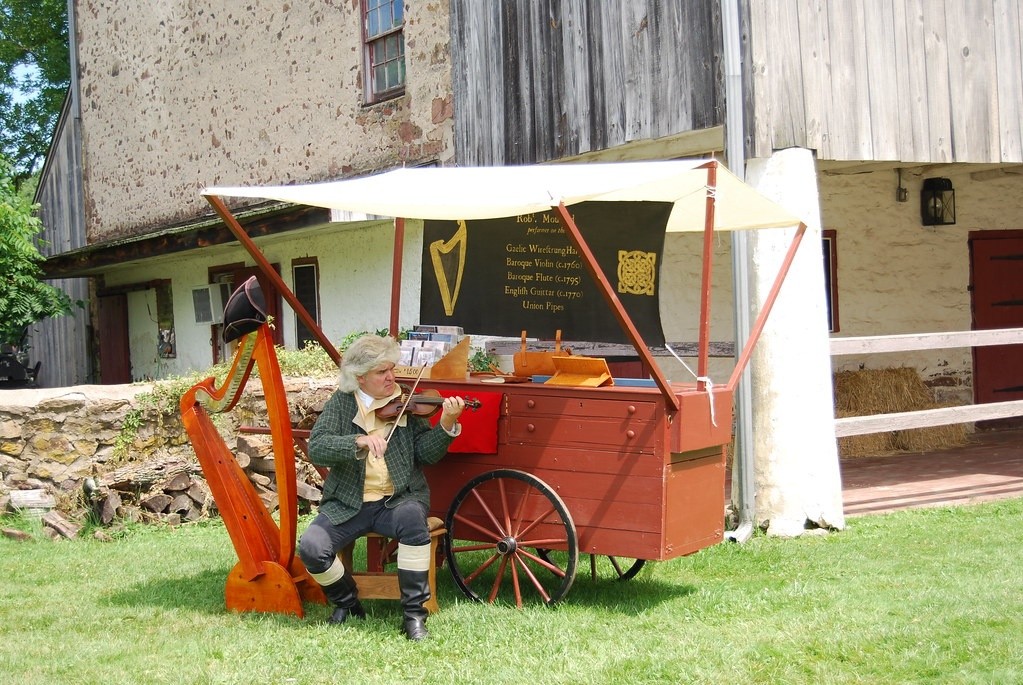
[191,283,232,326]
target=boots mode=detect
[319,565,365,623]
[396,567,431,640]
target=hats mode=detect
[221,276,267,344]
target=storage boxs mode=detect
[393,336,470,379]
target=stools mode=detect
[339,516,447,614]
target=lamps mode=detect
[921,177,956,226]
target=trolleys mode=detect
[238,361,737,612]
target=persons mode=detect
[299,334,465,641]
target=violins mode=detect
[374,387,482,422]
